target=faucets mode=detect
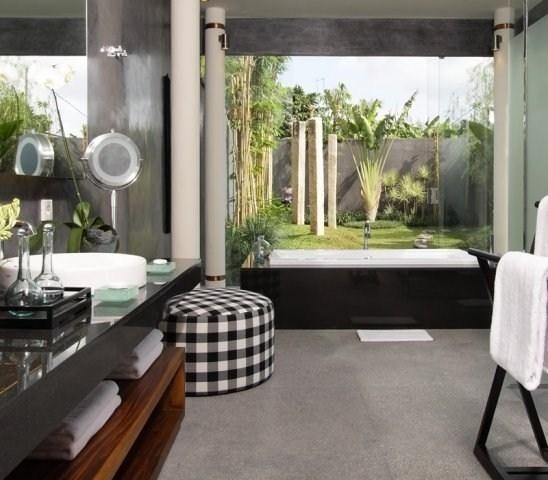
[0,216,35,261]
[362,219,372,250]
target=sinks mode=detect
[1,252,151,296]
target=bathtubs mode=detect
[239,247,498,331]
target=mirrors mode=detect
[13,135,54,177]
[0,1,90,180]
[85,129,145,189]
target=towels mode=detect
[114,328,164,366]
[529,194,548,252]
[30,394,123,459]
[488,250,548,392]
[107,341,164,379]
[27,379,120,443]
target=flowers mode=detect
[0,197,32,238]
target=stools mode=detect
[158,288,275,397]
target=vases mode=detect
[38,332,65,378]
[7,339,38,397]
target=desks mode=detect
[2,346,186,480]
